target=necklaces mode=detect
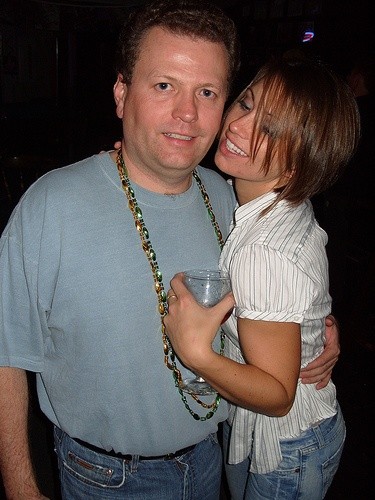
[115,148,228,420]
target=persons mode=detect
[100,51,362,500]
[0,1,342,500]
[223,37,375,354]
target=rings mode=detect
[165,294,178,302]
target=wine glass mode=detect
[181,269,233,395]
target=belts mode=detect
[71,438,197,461]
